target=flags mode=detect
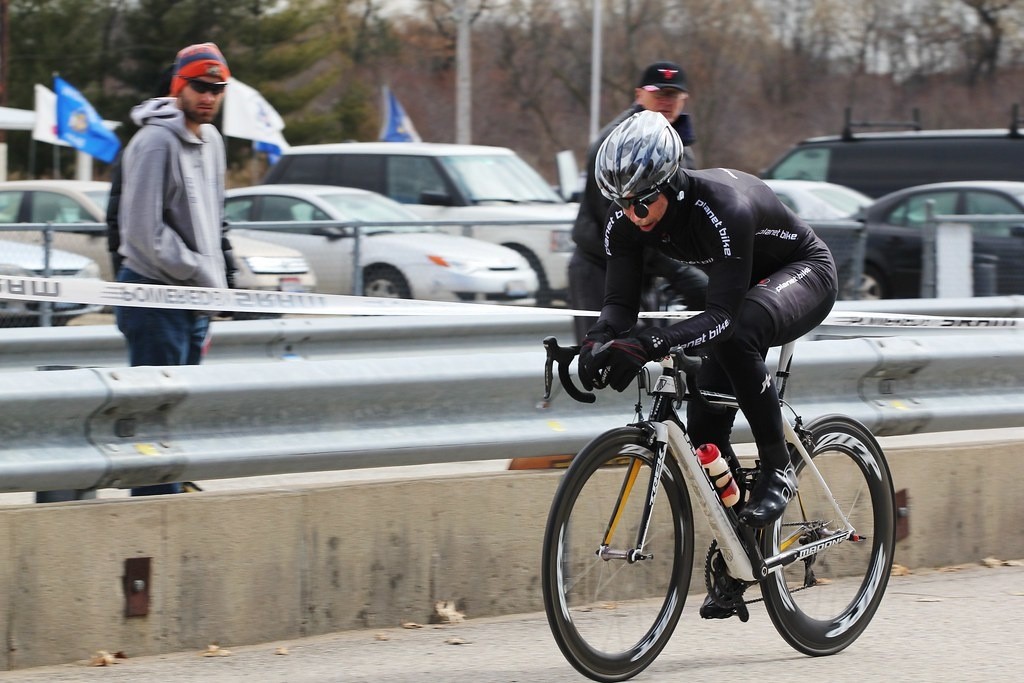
[31,78,122,163]
[221,75,290,163]
[376,89,423,143]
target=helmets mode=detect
[595,110,684,201]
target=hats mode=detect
[170,41,230,97]
[639,63,689,94]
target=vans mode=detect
[759,103,1024,200]
[849,181,1024,300]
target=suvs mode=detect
[259,142,582,309]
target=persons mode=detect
[578,111,840,618]
[110,41,229,499]
[567,63,696,344]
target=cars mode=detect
[224,184,540,309]
[0,179,318,321]
[0,239,106,328]
[762,179,921,224]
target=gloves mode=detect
[578,322,614,391]
[589,327,670,392]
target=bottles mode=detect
[696,443,741,509]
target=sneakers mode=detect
[700,553,746,617]
[739,461,799,528]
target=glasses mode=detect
[614,188,661,211]
[177,75,226,96]
[648,88,688,100]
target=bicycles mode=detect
[542,336,899,683]
